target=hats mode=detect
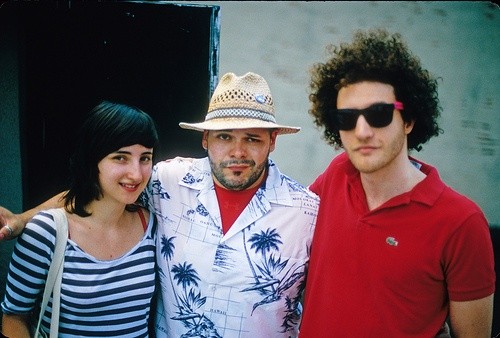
[177,73,302,136]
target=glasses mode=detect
[325,100,404,130]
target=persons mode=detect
[0,104,159,338]
[0,70,320,338]
[300,28,498,338]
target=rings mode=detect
[4,225,14,237]
